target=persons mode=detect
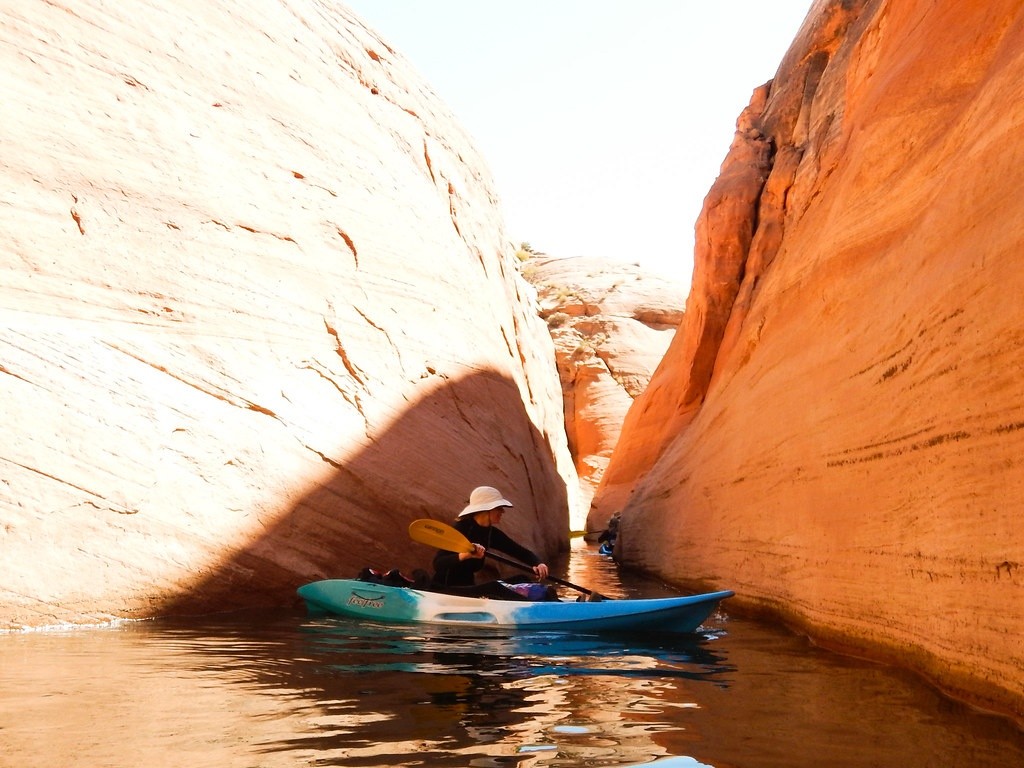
[598,511,622,550]
[431,486,602,602]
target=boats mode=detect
[295,578,735,635]
[599,543,612,554]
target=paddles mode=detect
[569,529,603,539]
[408,518,615,600]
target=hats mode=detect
[457,486,513,517]
[611,511,622,519]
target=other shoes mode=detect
[590,592,602,602]
[576,594,587,603]
